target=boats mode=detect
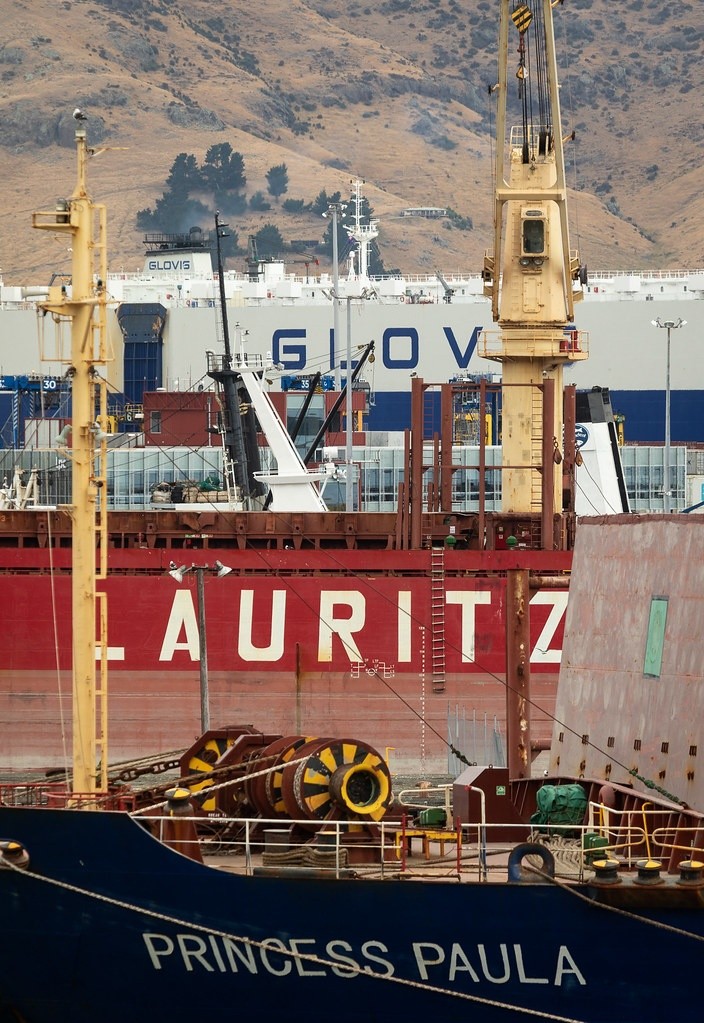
[0,107,704,1023]
[0,180,703,446]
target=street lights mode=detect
[321,202,347,392]
[323,286,379,513]
[651,316,687,514]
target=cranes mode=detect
[485,0,591,516]
